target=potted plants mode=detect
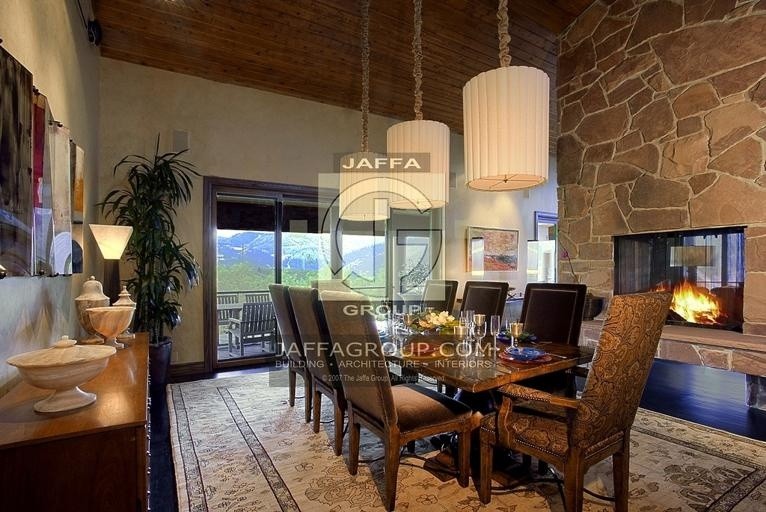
[101,131,204,389]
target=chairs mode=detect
[270,278,674,512]
[215,292,273,359]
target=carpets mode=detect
[166,362,766,512]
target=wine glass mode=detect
[509,322,524,347]
[504,319,515,348]
[454,309,501,361]
[403,313,414,335]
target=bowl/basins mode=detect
[584,297,604,320]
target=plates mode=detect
[504,347,548,360]
[400,341,446,359]
[496,332,537,344]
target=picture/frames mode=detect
[466,225,520,273]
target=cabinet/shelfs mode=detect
[0,332,153,512]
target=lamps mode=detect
[339,0,391,221]
[89,223,133,338]
[387,0,450,210]
[462,0,550,192]
